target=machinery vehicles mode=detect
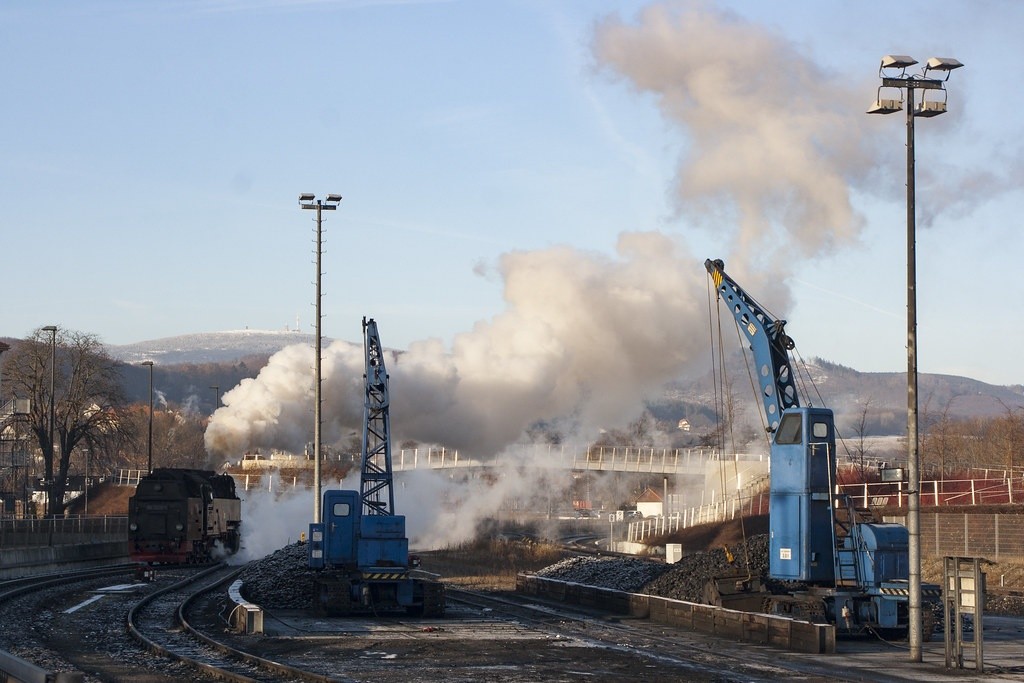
[308,315,446,617]
[704,259,943,643]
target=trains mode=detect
[127,466,242,564]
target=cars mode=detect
[575,509,593,516]
[627,511,643,517]
[662,512,681,522]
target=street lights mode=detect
[142,361,153,475]
[298,190,341,523]
[865,55,965,664]
[210,386,218,409]
[42,326,57,518]
[82,449,89,514]
